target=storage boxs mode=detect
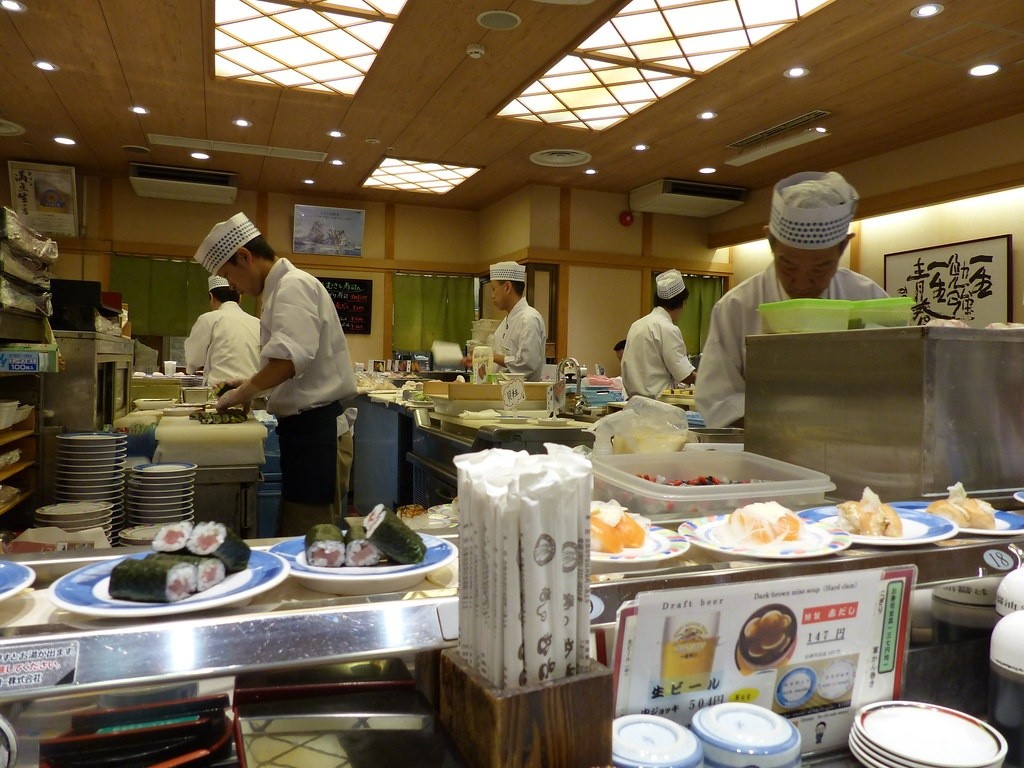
[0,347,50,372]
[7,343,58,373]
[592,447,836,530]
[437,649,614,768]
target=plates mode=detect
[775,666,817,708]
[160,407,203,416]
[0,533,458,617]
[589,489,1024,563]
[817,658,854,699]
[848,700,1009,768]
[735,638,796,676]
[33,432,198,547]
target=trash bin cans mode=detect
[251,409,285,538]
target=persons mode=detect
[695,171,892,429]
[612,339,627,361]
[620,269,697,402]
[460,260,547,382]
[184,274,262,407]
[194,211,358,538]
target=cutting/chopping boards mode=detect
[155,416,266,441]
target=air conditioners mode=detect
[129,162,241,204]
[630,179,748,219]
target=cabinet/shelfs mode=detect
[0,301,46,545]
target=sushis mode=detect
[108,520,251,603]
[729,501,800,541]
[926,494,997,530]
[833,499,903,537]
[589,504,645,553]
[305,502,426,567]
[189,382,248,423]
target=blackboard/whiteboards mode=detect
[315,277,373,335]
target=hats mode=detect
[656,269,686,299]
[768,172,859,250]
[490,261,526,281]
[193,212,261,277]
[208,275,229,291]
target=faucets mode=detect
[555,357,585,416]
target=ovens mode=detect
[406,411,597,511]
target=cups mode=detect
[738,602,797,669]
[660,608,720,697]
[164,360,177,376]
